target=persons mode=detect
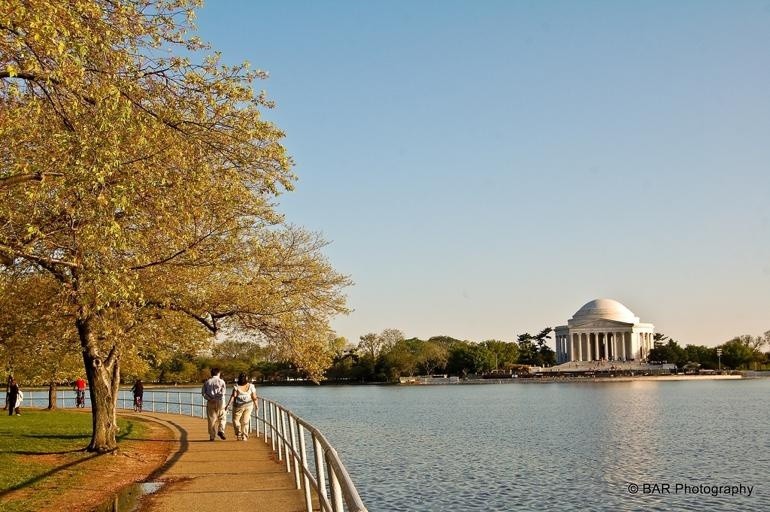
[130,379,143,406]
[8,379,22,416]
[201,366,227,440]
[225,373,259,442]
[73,375,87,406]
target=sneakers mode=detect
[236,432,242,440]
[242,434,248,441]
[217,431,226,440]
[210,436,215,441]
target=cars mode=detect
[483,371,547,379]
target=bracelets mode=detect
[228,403,230,405]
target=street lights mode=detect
[717,348,724,372]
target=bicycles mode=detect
[71,387,86,409]
[130,388,144,412]
